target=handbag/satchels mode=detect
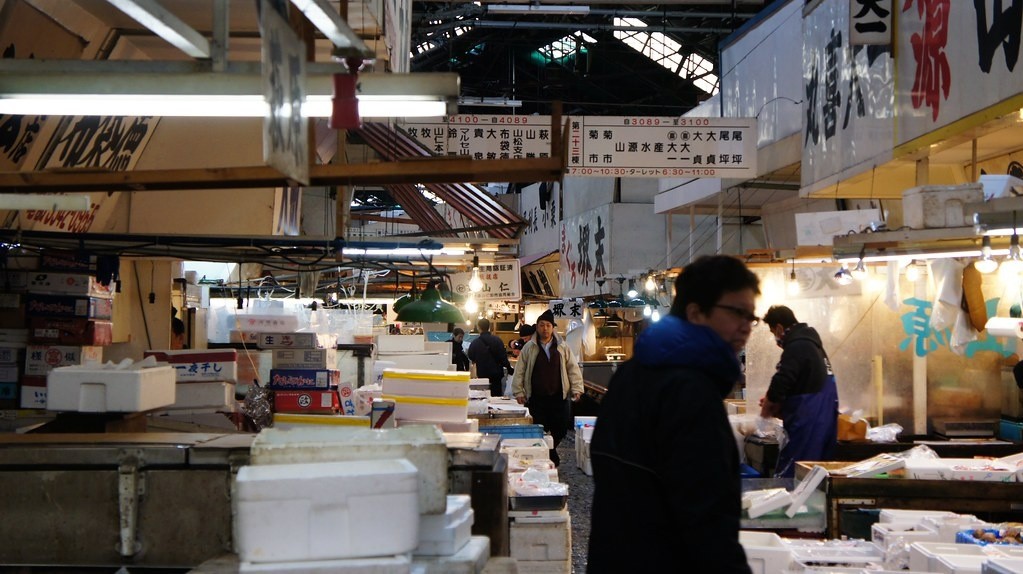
[504,375,515,398]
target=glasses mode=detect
[697,303,760,328]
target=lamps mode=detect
[589,299,596,308]
[629,298,646,307]
[607,277,627,308]
[605,323,619,327]
[0,58,464,117]
[606,308,624,322]
[327,57,364,130]
[392,256,467,325]
[623,278,640,308]
[592,280,610,319]
[974,236,997,274]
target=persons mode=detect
[758,306,839,478]
[584,253,762,574]
[170,317,188,350]
[445,319,536,397]
[512,309,584,467]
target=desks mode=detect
[744,436,778,476]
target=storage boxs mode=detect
[382,367,471,398]
[0,248,116,409]
[382,394,468,422]
[45,365,177,413]
[468,174,1023,574]
[249,426,448,514]
[144,349,239,382]
[233,457,421,562]
[256,332,316,349]
[274,391,342,415]
[269,369,341,390]
[208,314,296,394]
[172,381,237,408]
[271,348,328,370]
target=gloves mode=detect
[508,368,514,375]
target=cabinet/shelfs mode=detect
[0,433,509,574]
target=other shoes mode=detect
[550,448,559,467]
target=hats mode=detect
[520,325,534,337]
[539,309,555,328]
